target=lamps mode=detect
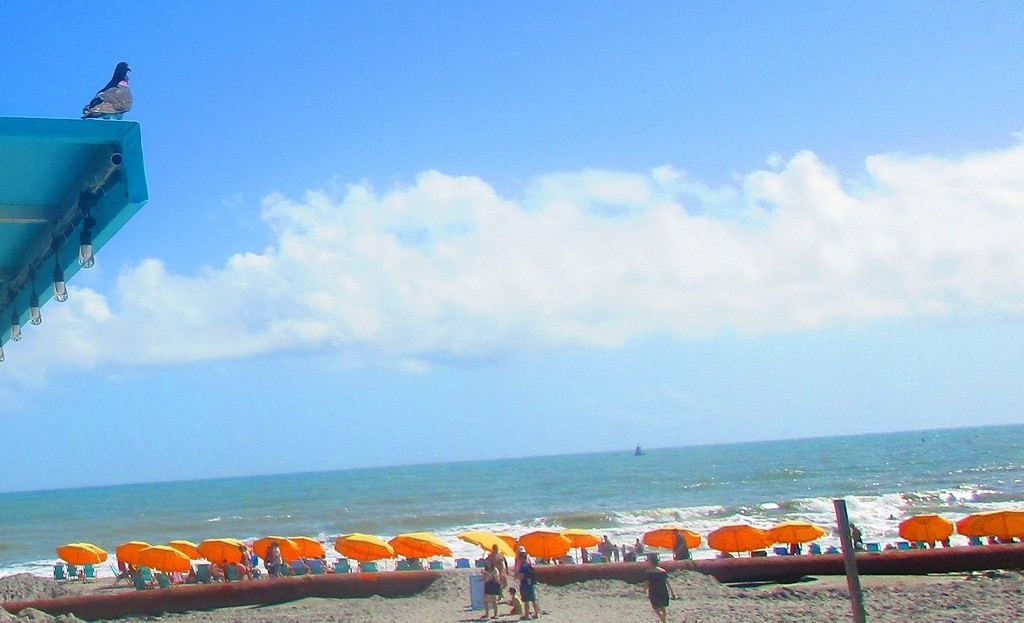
[9,304,22,342]
[28,291,42,325]
[52,265,69,302]
[0,338,5,362]
[77,229,95,268]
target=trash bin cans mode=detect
[469,574,495,610]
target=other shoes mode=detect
[520,616,531,620]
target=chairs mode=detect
[456,558,470,568]
[644,553,660,561]
[196,565,211,583]
[971,536,982,547]
[863,543,881,553]
[773,547,789,556]
[810,543,821,554]
[395,559,443,571]
[332,557,353,573]
[54,563,97,582]
[896,540,923,550]
[476,560,484,566]
[827,548,838,553]
[227,562,242,582]
[751,551,767,557]
[590,553,603,562]
[290,558,327,575]
[109,563,171,589]
[361,561,379,572]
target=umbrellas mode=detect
[58,531,453,579]
[564,528,604,559]
[643,525,703,559]
[458,528,519,559]
[516,530,572,564]
[899,514,954,546]
[708,520,825,558]
[956,509,1024,540]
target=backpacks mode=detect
[524,556,537,585]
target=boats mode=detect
[634,445,645,455]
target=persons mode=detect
[645,554,676,623]
[911,536,1024,549]
[485,544,508,599]
[581,535,620,564]
[481,558,502,619]
[622,544,626,562]
[787,542,802,555]
[847,522,863,548]
[503,586,522,614]
[514,545,532,579]
[271,542,287,575]
[635,538,643,554]
[516,554,538,620]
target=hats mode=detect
[519,546,526,553]
